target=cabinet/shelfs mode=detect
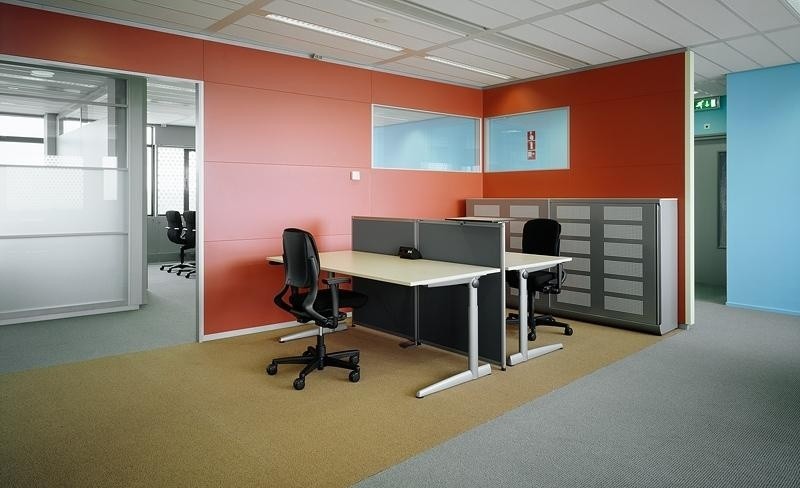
[465,197,680,337]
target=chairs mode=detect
[159,209,197,279]
[505,216,572,340]
[267,227,369,391]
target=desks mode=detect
[267,242,501,398]
[504,246,573,367]
